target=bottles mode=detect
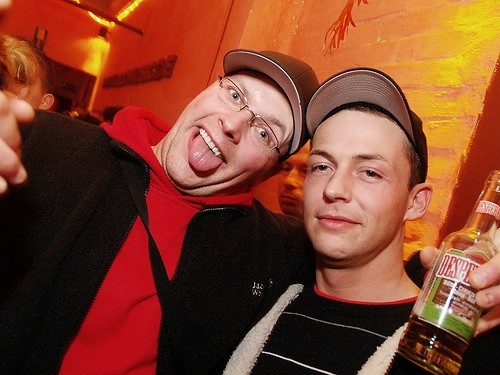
[398,170,500,375]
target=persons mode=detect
[1,36,55,111]
[0,49,500,374]
[221,67,500,375]
[278,137,311,219]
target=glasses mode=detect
[217,74,284,159]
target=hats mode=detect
[223,48,319,159]
[306,67,428,184]
